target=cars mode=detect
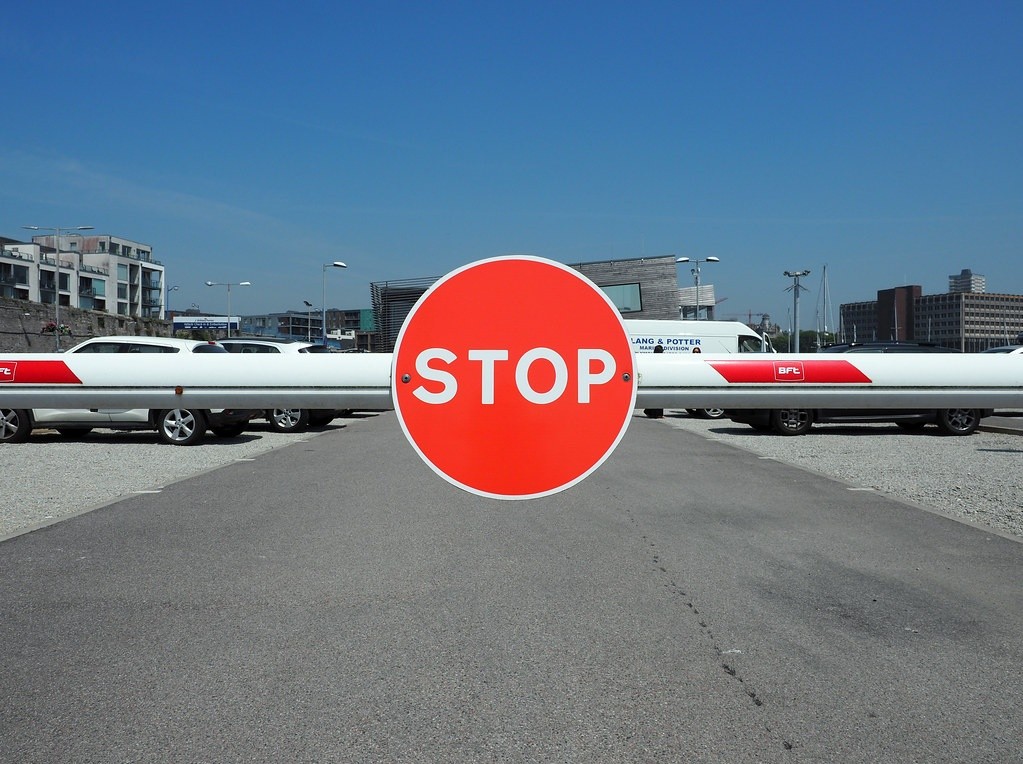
[978,344,1023,354]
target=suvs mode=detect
[727,339,994,436]
[0,334,262,446]
[215,337,337,434]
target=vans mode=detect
[624,317,780,420]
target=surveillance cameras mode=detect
[805,270,811,273]
[784,271,789,276]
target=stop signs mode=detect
[388,252,642,502]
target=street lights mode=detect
[205,280,251,338]
[191,302,201,317]
[320,261,348,345]
[20,225,97,348]
[166,285,180,321]
[674,256,721,321]
[304,300,314,342]
